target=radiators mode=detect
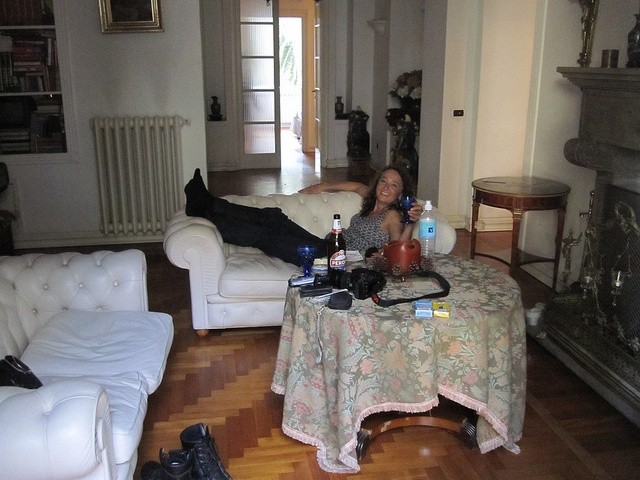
[90,113,186,235]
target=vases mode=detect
[334,95,345,114]
[210,94,222,116]
[390,147,418,197]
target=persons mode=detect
[184,168,421,270]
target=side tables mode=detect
[471,177,571,297]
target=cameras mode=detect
[328,267,387,300]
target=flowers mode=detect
[390,66,421,146]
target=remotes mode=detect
[301,284,332,293]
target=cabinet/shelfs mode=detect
[0,2,68,156]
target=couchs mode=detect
[1,248,175,480]
[162,190,458,338]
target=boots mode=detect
[140,448,195,479]
[180,423,232,480]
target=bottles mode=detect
[419,200,435,262]
[328,214,347,275]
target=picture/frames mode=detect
[98,2,164,34]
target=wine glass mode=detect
[297,248,316,278]
[399,196,415,224]
